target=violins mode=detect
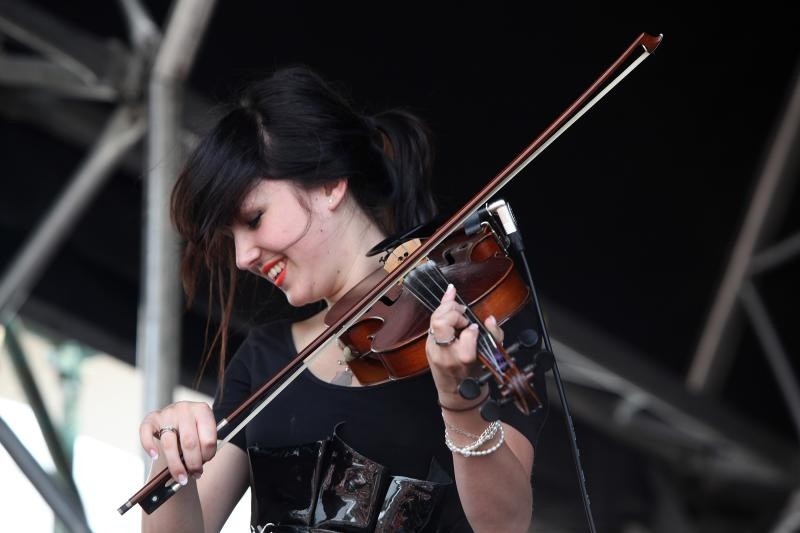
[324,222,557,424]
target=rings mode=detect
[159,423,178,436]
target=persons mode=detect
[141,64,550,533]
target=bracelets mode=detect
[437,391,490,412]
[442,413,504,457]
